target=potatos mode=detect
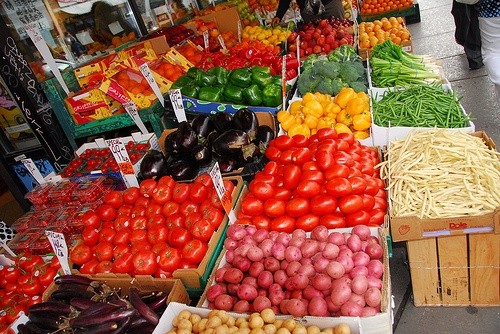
[168,309,352,334]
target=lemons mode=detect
[242,26,291,49]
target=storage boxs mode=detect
[2,0,498,334]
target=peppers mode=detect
[277,88,372,140]
[371,80,478,128]
[171,44,298,106]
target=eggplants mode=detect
[18,274,168,334]
[140,107,274,181]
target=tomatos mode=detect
[0,178,113,333]
[71,174,234,278]
[236,127,386,231]
[63,140,145,177]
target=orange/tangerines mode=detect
[81,60,183,117]
[361,0,413,14]
[359,16,409,48]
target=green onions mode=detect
[368,38,444,86]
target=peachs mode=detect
[287,19,354,59]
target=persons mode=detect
[451,0,484,70]
[69,0,190,58]
[271,0,345,28]
[479,0,500,103]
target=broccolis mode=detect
[296,45,368,96]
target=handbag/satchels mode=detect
[451,1,480,50]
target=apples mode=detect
[159,19,241,52]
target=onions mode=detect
[207,225,383,315]
